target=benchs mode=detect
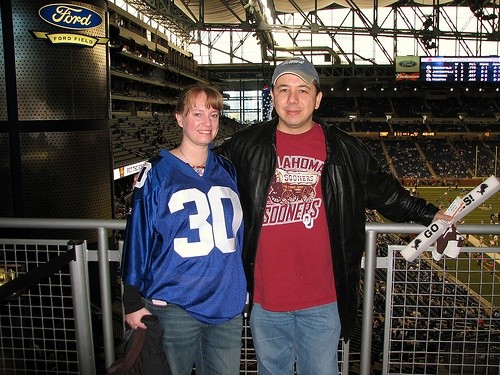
[111,115,243,167]
[359,209,500,375]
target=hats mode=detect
[271,56,320,86]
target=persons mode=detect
[133,57,453,375]
[121,86,247,375]
[364,135,500,361]
